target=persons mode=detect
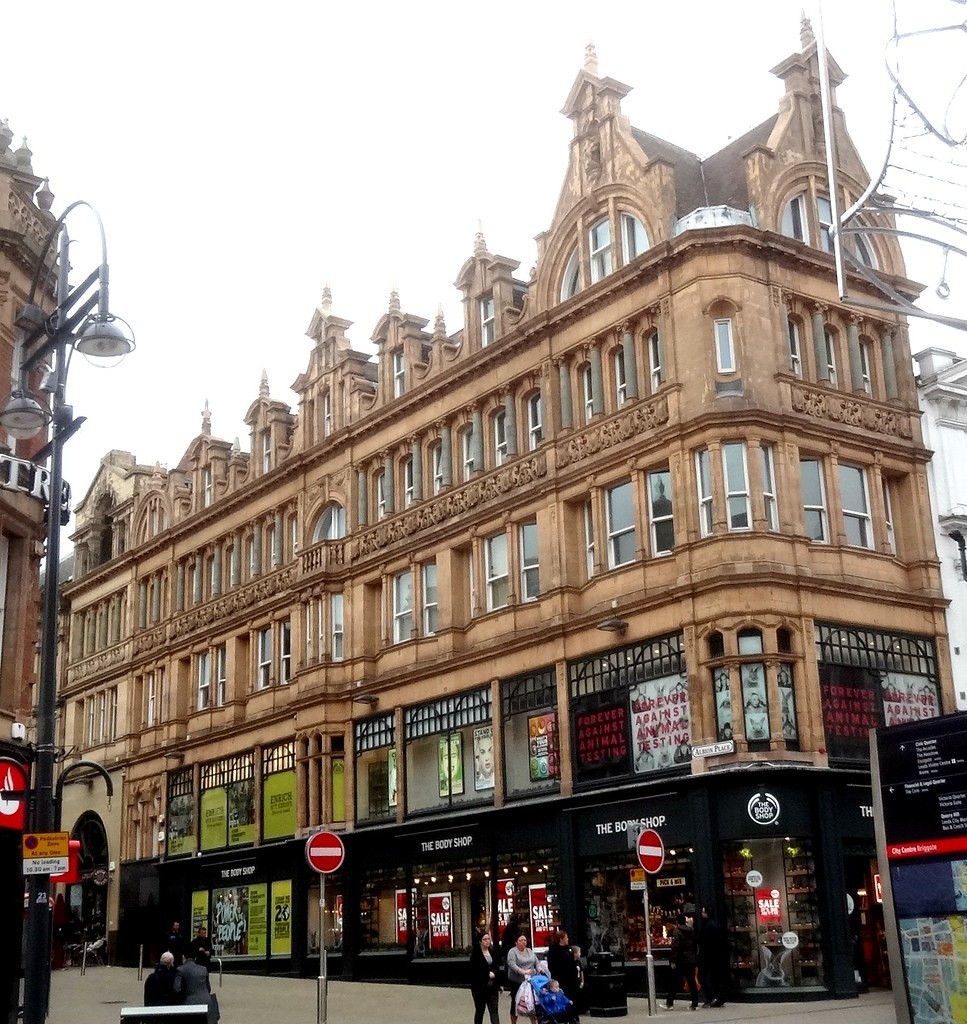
[188,928,216,972]
[470,933,504,1024]
[744,691,767,713]
[778,671,792,687]
[506,934,545,1024]
[543,980,580,1024]
[143,952,183,1008]
[717,673,730,692]
[695,904,728,1008]
[546,931,586,1013]
[440,741,462,795]
[173,948,217,1024]
[657,914,700,1011]
[163,918,187,965]
[475,736,495,789]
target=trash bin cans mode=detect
[584,950,629,1017]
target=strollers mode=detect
[527,970,580,1024]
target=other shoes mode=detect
[687,1006,699,1011]
[658,1003,673,1010]
[702,999,717,1008]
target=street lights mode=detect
[0,200,136,1024]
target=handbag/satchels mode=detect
[684,966,701,992]
[514,980,535,1017]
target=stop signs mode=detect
[305,832,345,874]
[636,829,665,874]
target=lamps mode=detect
[597,620,630,633]
[41,502,73,527]
[353,694,379,705]
[164,752,186,759]
